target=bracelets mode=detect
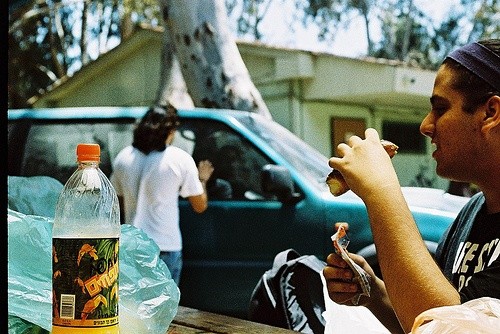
[201,178,208,185]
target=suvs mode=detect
[5,107,479,331]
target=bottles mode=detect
[51,146,120,334]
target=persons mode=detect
[109,103,216,290]
[323,38,500,334]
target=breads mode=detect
[326,140,400,199]
